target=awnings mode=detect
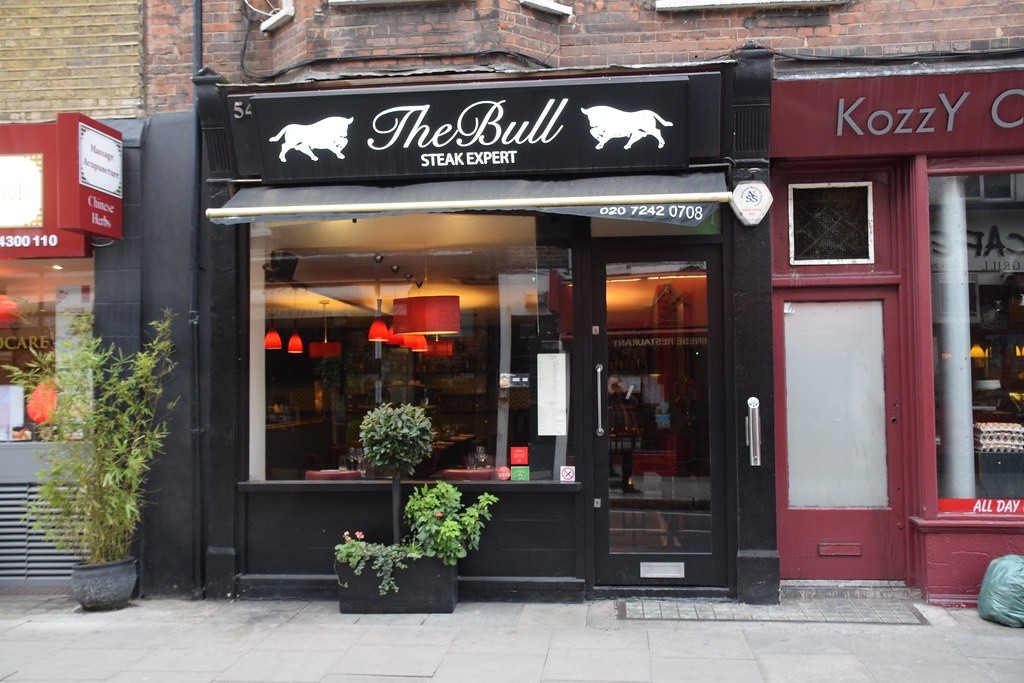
[206,172,734,227]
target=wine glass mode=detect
[346,447,366,473]
[475,446,487,471]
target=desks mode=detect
[407,434,476,479]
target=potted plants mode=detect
[0,306,181,611]
[333,403,499,613]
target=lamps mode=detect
[368,255,389,342]
[423,334,453,357]
[412,281,428,352]
[400,274,416,348]
[265,275,283,350]
[729,168,774,227]
[386,265,405,345]
[288,287,303,354]
[309,300,341,358]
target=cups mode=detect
[485,455,493,469]
[339,455,348,472]
[466,452,476,470]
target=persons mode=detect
[607,373,642,495]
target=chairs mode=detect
[305,470,361,480]
[443,468,495,481]
